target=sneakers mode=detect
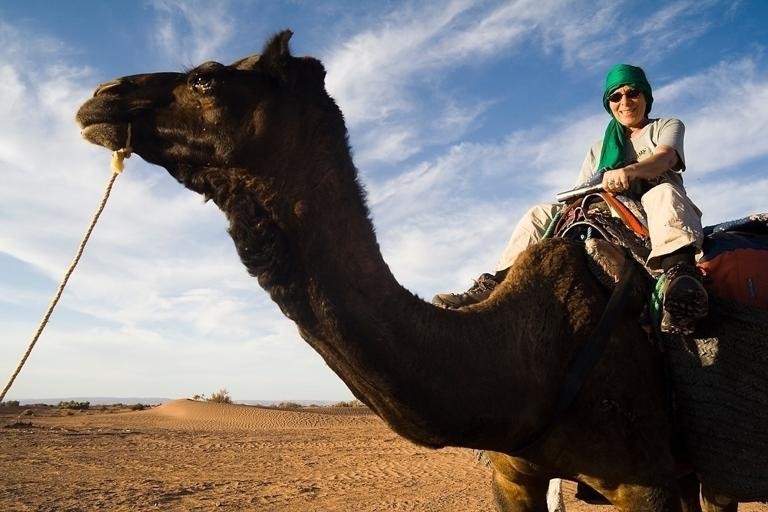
[659,265,712,338]
[432,272,501,309]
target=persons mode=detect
[429,64,710,337]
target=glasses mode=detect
[608,88,643,103]
